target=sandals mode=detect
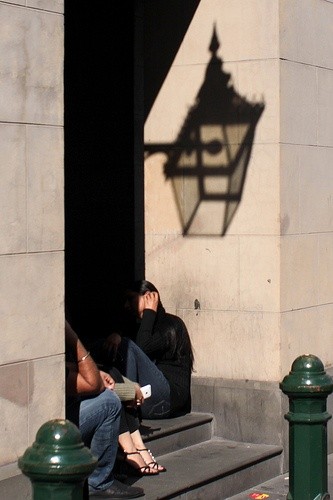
[122,449,165,475]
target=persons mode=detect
[109,279,190,418]
[67,314,164,474]
[63,319,147,500]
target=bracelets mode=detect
[74,351,90,366]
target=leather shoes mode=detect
[91,480,145,498]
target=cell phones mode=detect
[140,384,151,400]
